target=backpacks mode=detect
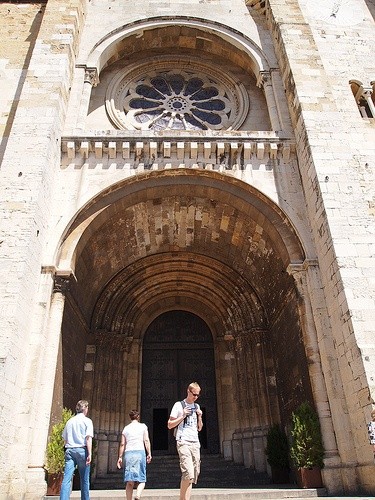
[173,400,199,438]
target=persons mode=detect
[116,409,152,500]
[168,382,203,500]
[60,400,94,500]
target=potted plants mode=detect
[288,399,323,489]
[46,407,98,497]
[263,422,290,484]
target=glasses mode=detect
[190,390,200,397]
